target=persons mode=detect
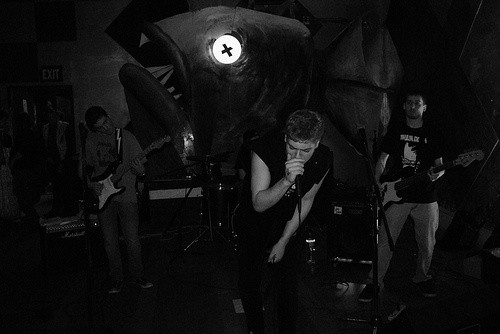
[359,90,446,302]
[79,105,153,294]
[237,109,333,334]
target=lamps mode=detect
[212,32,242,65]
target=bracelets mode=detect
[136,172,146,177]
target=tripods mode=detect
[185,158,230,250]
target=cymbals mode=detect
[184,152,222,160]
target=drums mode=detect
[211,184,230,227]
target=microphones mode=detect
[296,174,303,196]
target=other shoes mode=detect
[359,283,380,302]
[137,278,154,288]
[415,280,436,298]
[108,281,121,293]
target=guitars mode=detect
[81,140,162,214]
[372,150,483,216]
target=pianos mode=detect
[145,182,203,201]
